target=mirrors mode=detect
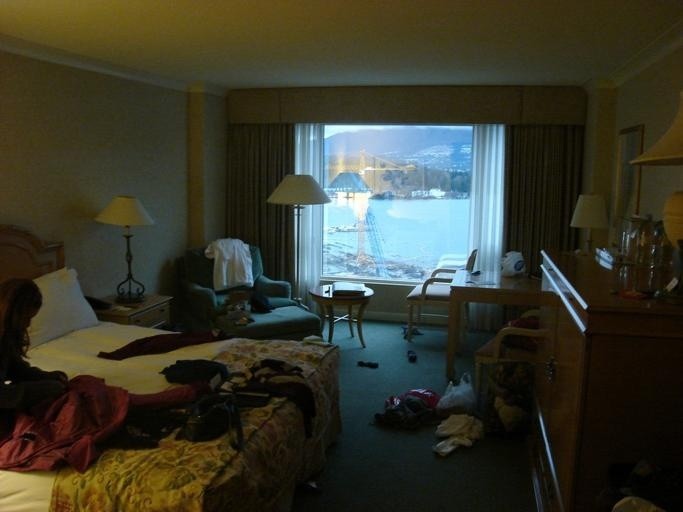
[613,124,644,219]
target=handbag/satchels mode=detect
[175,392,244,451]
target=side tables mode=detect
[308,285,374,349]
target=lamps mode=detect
[569,193,608,253]
[628,104,683,249]
[94,195,155,302]
[329,171,372,273]
[266,175,331,310]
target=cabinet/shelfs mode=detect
[532,249,683,512]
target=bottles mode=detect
[620,219,677,301]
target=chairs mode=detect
[406,249,477,341]
[177,245,322,341]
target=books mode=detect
[331,282,367,300]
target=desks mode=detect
[446,269,541,381]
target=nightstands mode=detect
[95,293,173,329]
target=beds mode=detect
[1,224,343,511]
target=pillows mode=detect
[22,266,99,350]
